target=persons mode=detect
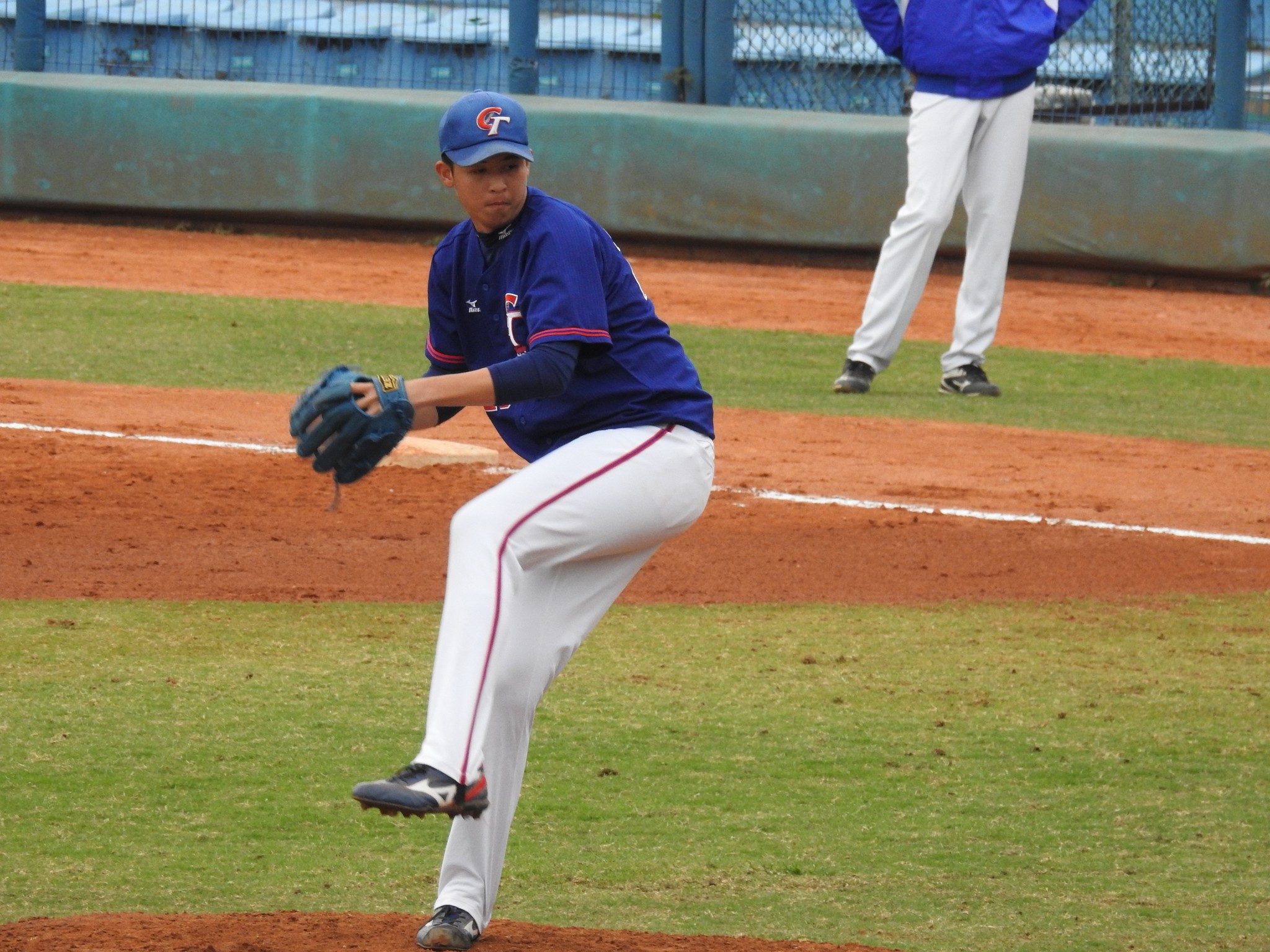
[288,88,715,952]
[833,0,1094,397]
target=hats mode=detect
[438,89,534,167]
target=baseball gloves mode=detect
[287,363,413,484]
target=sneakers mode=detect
[835,361,873,393]
[939,363,1000,395]
[352,764,490,819]
[416,904,480,951]
